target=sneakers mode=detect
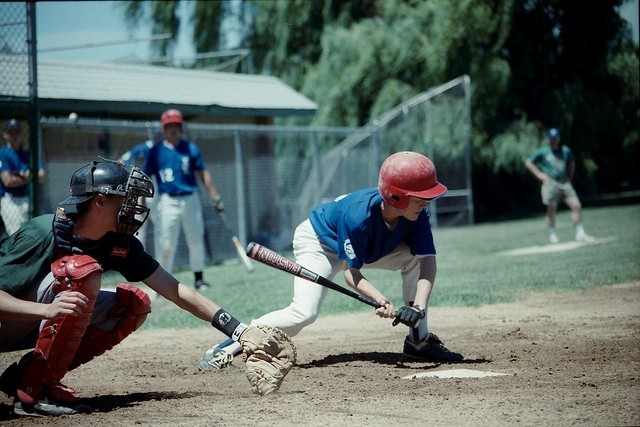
[0,361,30,398]
[198,342,234,371]
[12,385,92,417]
[404,331,464,362]
[575,233,594,242]
[551,233,559,243]
[196,279,211,291]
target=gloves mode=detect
[392,300,425,327]
[213,197,224,212]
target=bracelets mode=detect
[211,308,249,343]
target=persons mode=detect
[0,161,298,419]
[200,151,464,375]
[524,127,596,244]
[143,108,225,290]
[0,117,46,240]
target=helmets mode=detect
[58,156,155,239]
[161,109,183,133]
[3,119,21,131]
[378,151,448,209]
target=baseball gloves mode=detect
[239,323,297,400]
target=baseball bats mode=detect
[212,200,253,274]
[246,242,420,328]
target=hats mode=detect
[548,127,559,136]
